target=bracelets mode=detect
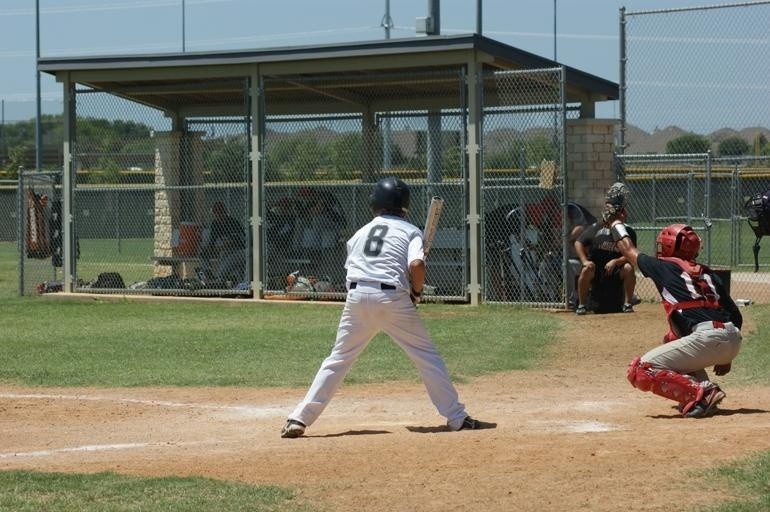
[411,287,423,297]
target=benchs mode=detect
[149,227,471,290]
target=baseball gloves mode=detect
[602,182,630,225]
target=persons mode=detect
[280,178,497,438]
[601,202,742,418]
[197,186,348,292]
[574,203,637,316]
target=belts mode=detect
[350,281,396,290]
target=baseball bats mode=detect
[497,241,506,301]
[507,248,535,300]
[416,196,443,255]
[518,247,555,302]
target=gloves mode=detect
[410,288,423,306]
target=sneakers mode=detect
[622,304,634,313]
[280,419,306,438]
[575,305,586,315]
[682,384,726,420]
[447,416,480,430]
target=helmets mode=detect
[367,178,411,211]
[743,191,770,236]
[655,224,704,261]
